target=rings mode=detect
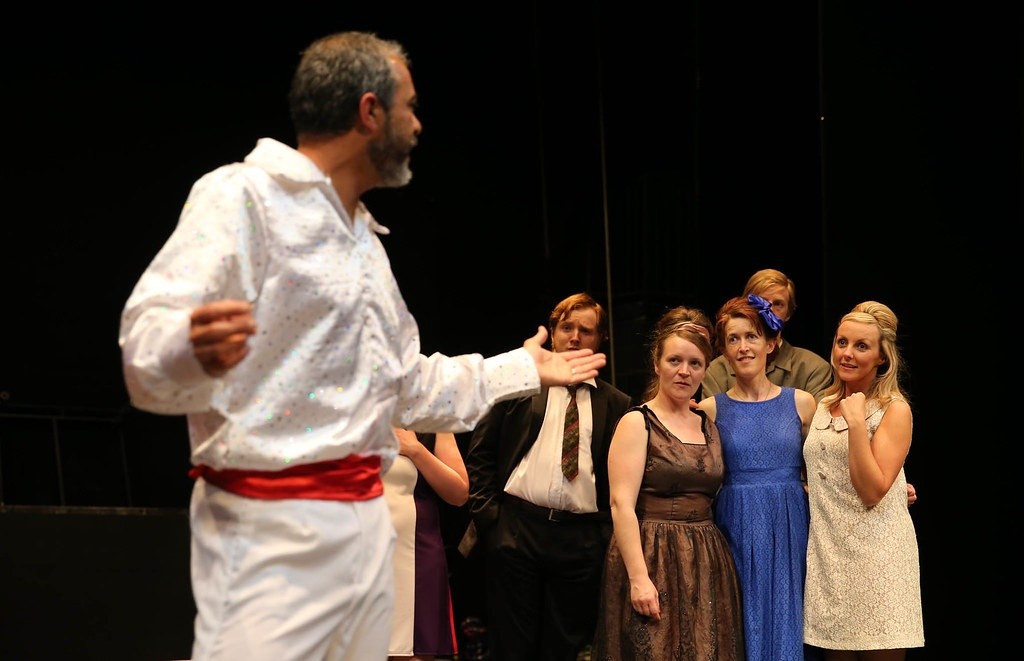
[572,368,576,374]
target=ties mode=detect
[562,384,584,481]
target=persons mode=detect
[697,295,918,661]
[609,308,743,661]
[799,301,925,661]
[378,427,470,661]
[120,29,607,661]
[700,268,837,485]
[461,293,633,661]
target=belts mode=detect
[501,495,600,523]
